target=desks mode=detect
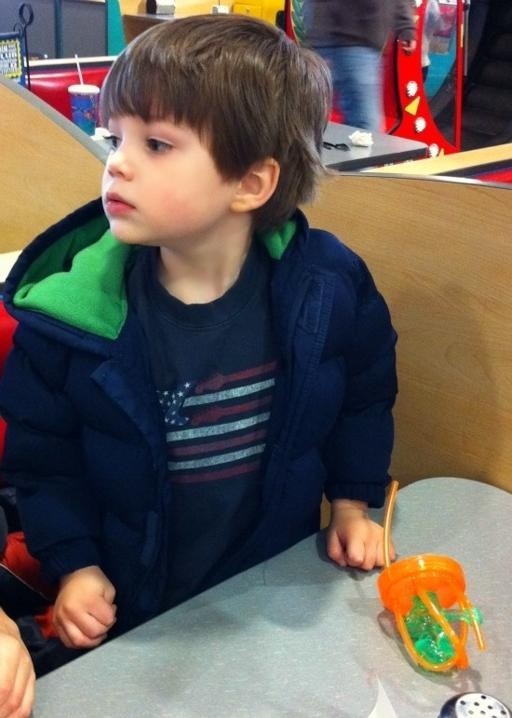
[24,475,510,718]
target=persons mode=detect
[301,0,417,134]
[0,14,398,650]
[0,506,36,718]
[422,0,440,83]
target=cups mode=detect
[68,84,100,136]
